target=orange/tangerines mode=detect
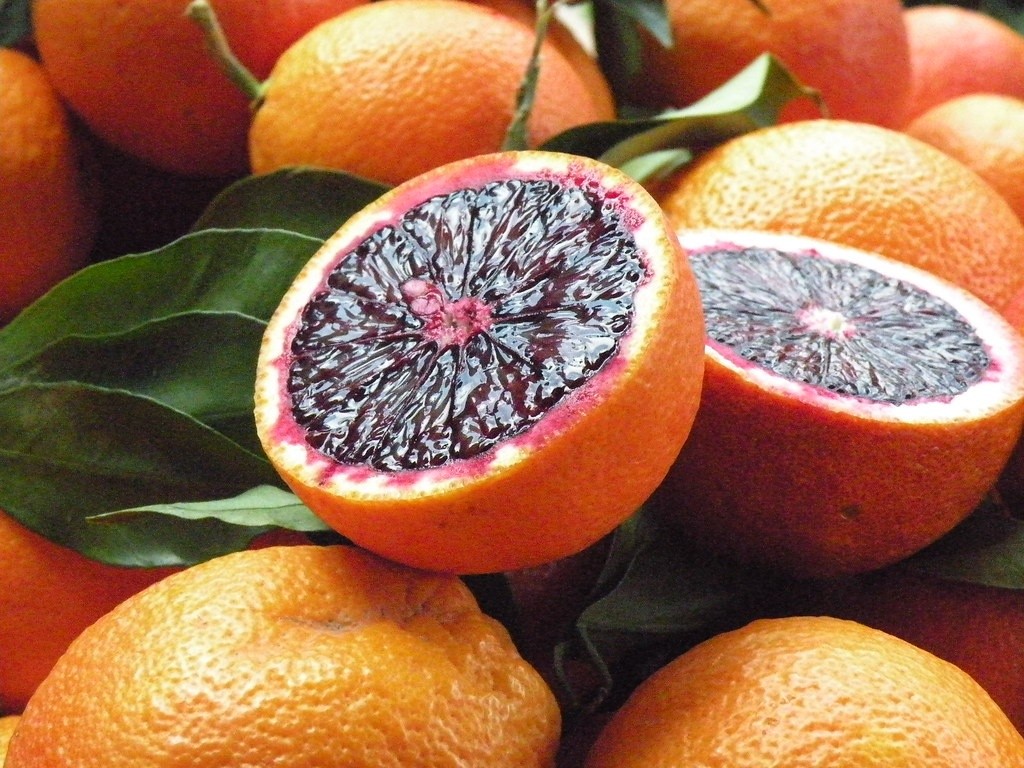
[0,0,1024,766]
